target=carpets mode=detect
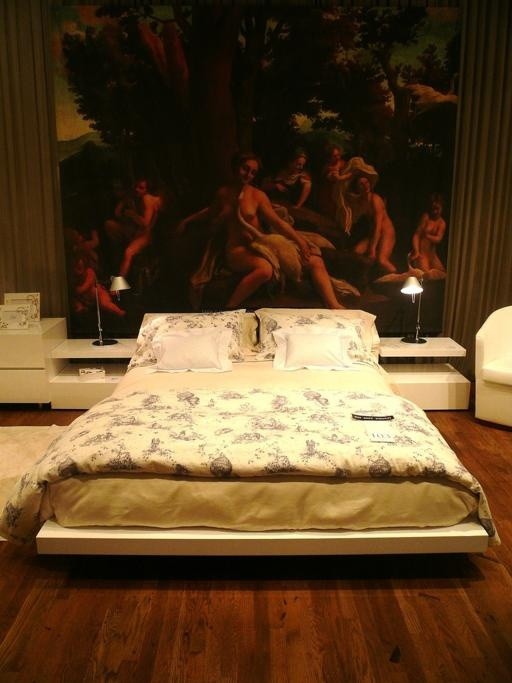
[0,426,68,542]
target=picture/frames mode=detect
[0,305,29,329]
[4,293,40,322]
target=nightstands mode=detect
[379,337,471,411]
[50,338,136,410]
[0,318,67,408]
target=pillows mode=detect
[154,328,233,373]
[252,307,377,367]
[129,313,257,367]
[270,325,357,371]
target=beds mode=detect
[1,308,501,556]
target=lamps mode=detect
[93,275,131,346]
[400,276,427,344]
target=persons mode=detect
[173,144,398,310]
[69,255,126,316]
[409,199,446,273]
[66,228,100,267]
[103,178,159,279]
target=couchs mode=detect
[474,306,512,427]
